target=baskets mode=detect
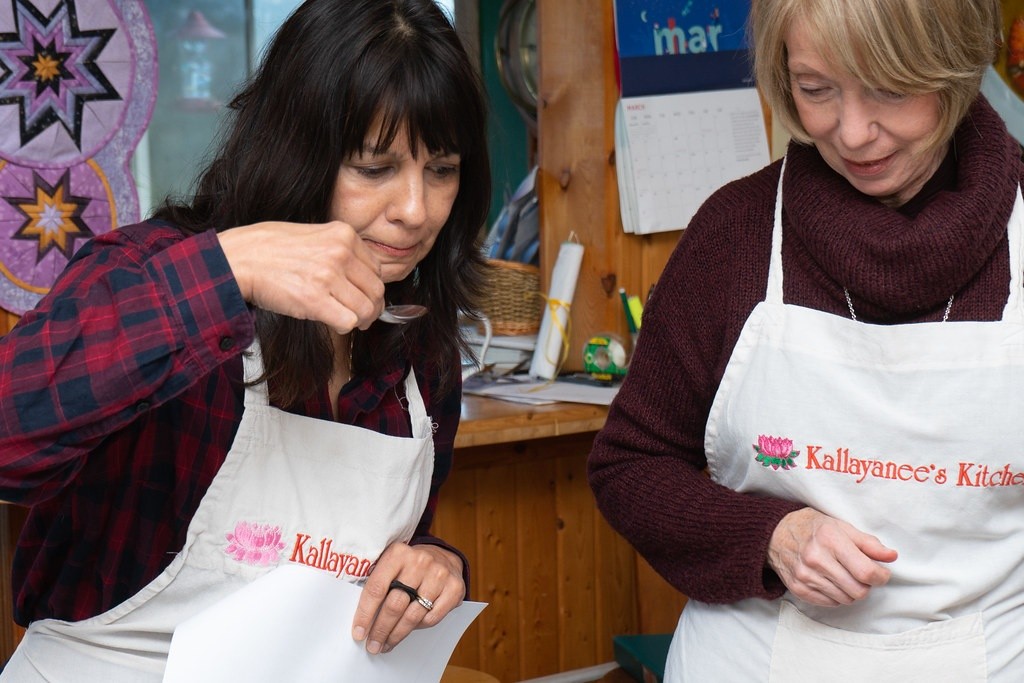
[475,258,542,336]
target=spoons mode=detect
[380,303,429,324]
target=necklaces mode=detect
[345,327,358,383]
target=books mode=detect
[454,325,541,366]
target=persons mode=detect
[587,2,1024,680]
[0,0,493,683]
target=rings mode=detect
[414,594,433,611]
[389,578,418,602]
